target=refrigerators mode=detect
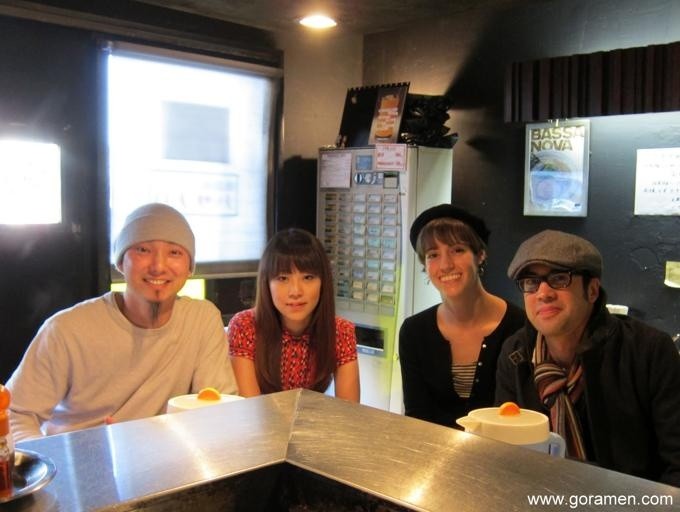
[312,141,455,419]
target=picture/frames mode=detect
[519,119,591,218]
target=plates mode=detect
[0,445,58,505]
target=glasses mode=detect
[516,267,584,293]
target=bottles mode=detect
[1,385,15,499]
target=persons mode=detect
[398,204,527,428]
[228,228,359,405]
[493,229,680,490]
[4,203,238,444]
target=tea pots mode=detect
[455,405,567,461]
[166,391,248,415]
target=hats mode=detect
[408,203,490,250]
[111,202,197,280]
[507,228,602,279]
